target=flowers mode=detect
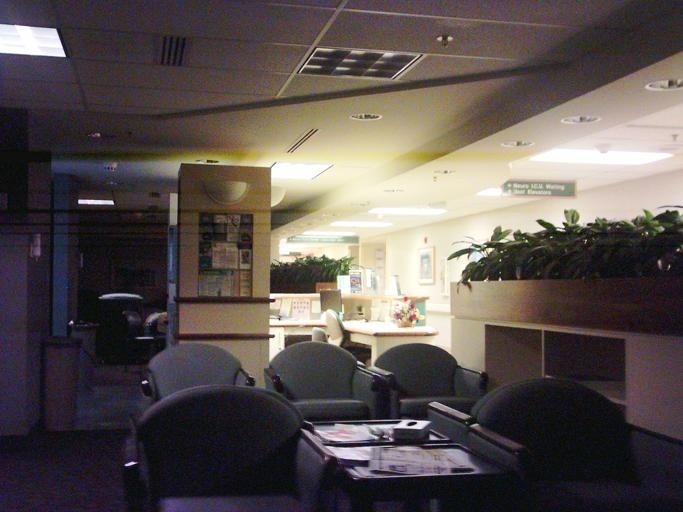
[392,295,424,324]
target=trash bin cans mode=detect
[98,293,143,365]
[41,335,82,432]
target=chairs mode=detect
[427,383,516,450]
[264,341,389,419]
[468,381,635,511]
[368,343,490,422]
[327,308,370,360]
[124,387,337,512]
[126,343,257,429]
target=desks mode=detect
[269,319,439,367]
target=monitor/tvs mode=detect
[320,289,342,315]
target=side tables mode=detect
[312,420,452,493]
[325,444,501,512]
